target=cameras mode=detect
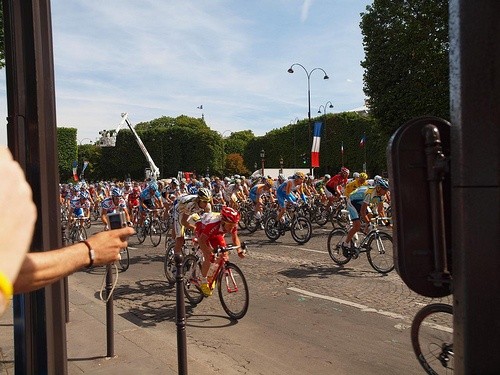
[106,211,106,224]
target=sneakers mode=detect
[197,275,215,296]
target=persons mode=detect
[0,145,138,375]
[55,167,393,297]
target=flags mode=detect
[360,137,365,147]
[311,122,323,166]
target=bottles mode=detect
[207,262,215,277]
[281,215,286,223]
[350,226,359,243]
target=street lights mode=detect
[260,149,265,177]
[279,156,283,176]
[77,138,93,173]
[318,101,334,142]
[288,63,329,175]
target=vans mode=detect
[251,168,313,181]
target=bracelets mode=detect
[0,273,13,302]
[79,240,94,268]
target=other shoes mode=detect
[341,242,349,258]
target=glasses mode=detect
[114,197,122,199]
[81,198,87,200]
[152,190,157,191]
[199,199,211,203]
[224,222,237,227]
[268,186,272,188]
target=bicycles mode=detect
[411,303,455,375]
[327,206,396,275]
[163,236,250,319]
[59,183,397,269]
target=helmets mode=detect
[221,206,240,223]
[374,175,382,181]
[359,173,368,178]
[325,174,331,179]
[59,174,293,199]
[353,172,360,178]
[305,175,308,180]
[376,178,390,189]
[341,167,350,175]
[198,188,211,200]
[294,171,304,180]
[309,176,314,181]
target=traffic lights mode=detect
[303,157,306,164]
